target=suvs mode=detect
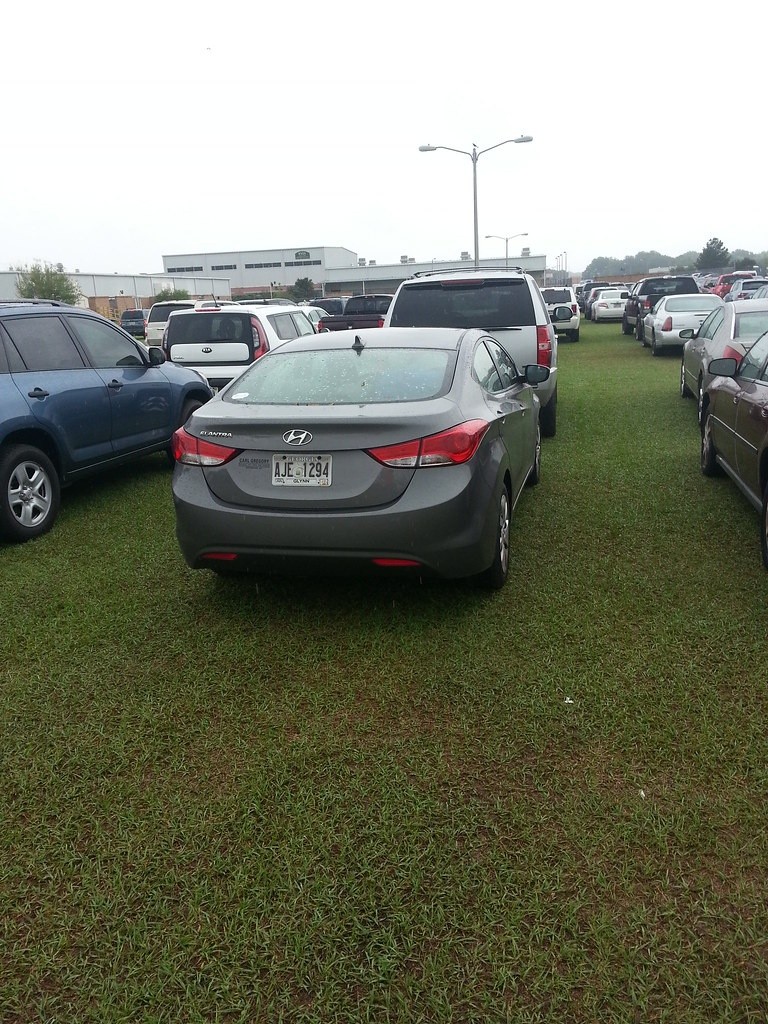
[382,267,558,437]
[538,287,582,342]
[0,298,219,541]
[620,276,701,340]
[119,308,148,339]
[711,274,754,299]
[723,279,768,302]
[309,295,353,317]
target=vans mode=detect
[145,299,242,349]
[732,271,758,277]
[162,304,332,388]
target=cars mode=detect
[574,282,638,313]
[698,331,768,565]
[745,285,768,299]
[293,307,333,334]
[177,328,552,593]
[693,273,718,294]
[643,294,727,356]
[584,287,619,320]
[678,298,768,401]
[591,290,632,324]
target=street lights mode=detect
[555,251,567,272]
[419,134,533,275]
[485,232,529,273]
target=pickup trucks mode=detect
[317,294,396,332]
